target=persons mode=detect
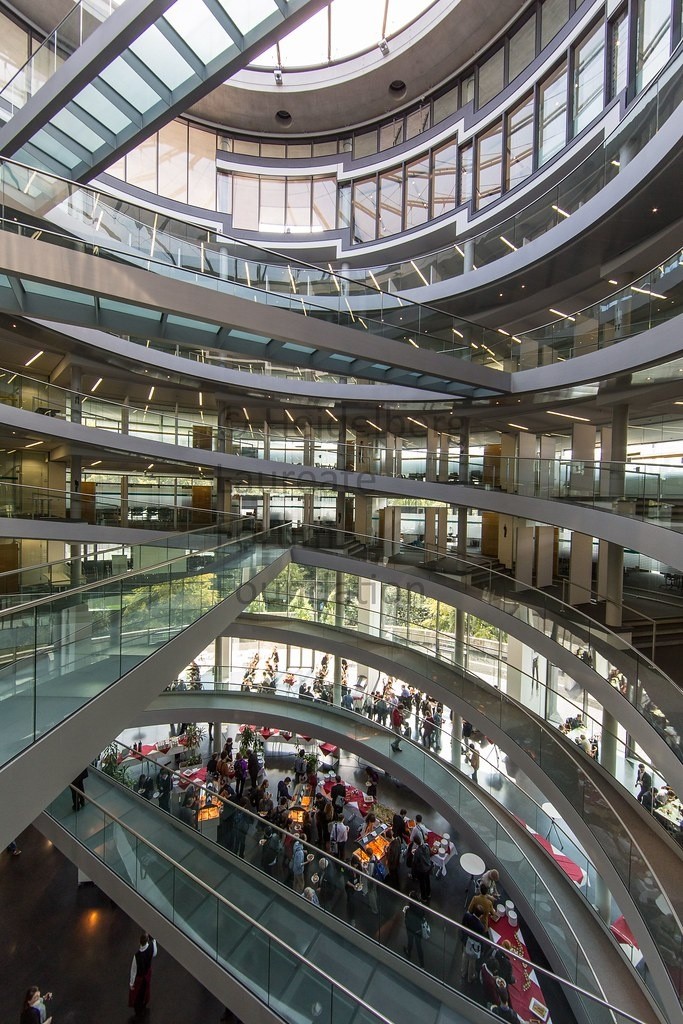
[7,840,22,855]
[575,648,628,697]
[129,933,158,1014]
[558,714,683,844]
[20,985,53,1024]
[135,648,522,1024]
[70,768,89,811]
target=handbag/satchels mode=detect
[299,773,308,783]
[465,936,481,959]
[421,917,431,940]
[335,795,346,807]
[330,840,338,854]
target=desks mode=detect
[234,729,341,773]
[487,911,553,1024]
[318,774,458,877]
[121,735,197,775]
[167,758,265,794]
[566,726,593,745]
[654,798,683,833]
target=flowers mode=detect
[282,672,297,687]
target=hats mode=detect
[496,987,509,1001]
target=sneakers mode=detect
[391,744,402,752]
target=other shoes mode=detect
[13,851,22,855]
[403,946,412,958]
[418,956,424,968]
[421,894,432,902]
[79,798,85,807]
[72,805,79,811]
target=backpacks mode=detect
[271,806,287,826]
[370,856,386,881]
[249,793,258,807]
[234,759,244,779]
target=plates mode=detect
[258,839,266,846]
[365,781,372,787]
[434,832,451,857]
[307,854,314,862]
[354,884,362,891]
[311,874,319,883]
[319,858,328,869]
[403,906,410,913]
[259,811,267,817]
[497,900,518,927]
[495,977,507,989]
[153,792,160,798]
[138,789,145,794]
[172,769,200,779]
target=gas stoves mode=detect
[324,770,336,782]
